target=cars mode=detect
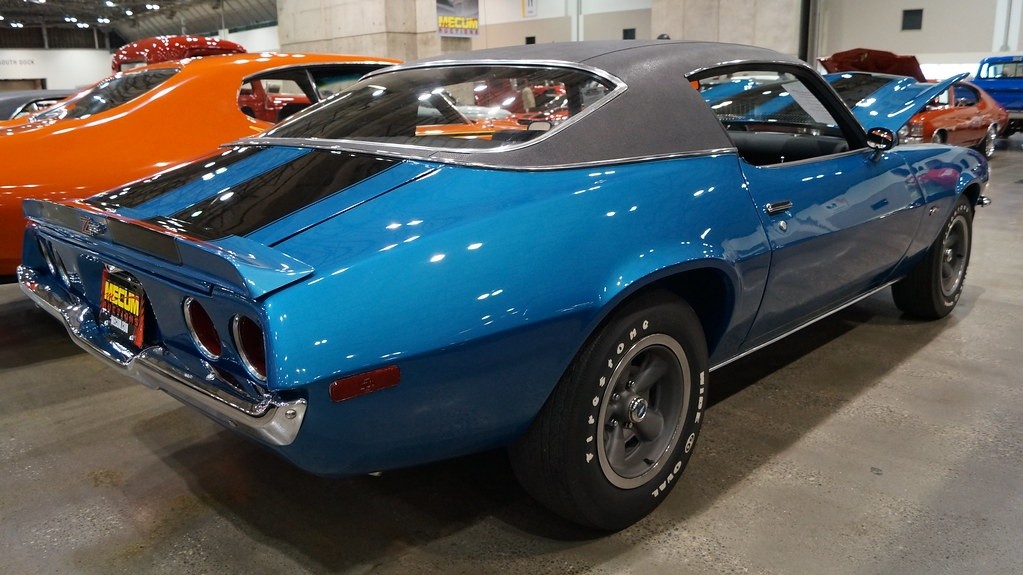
[797,46,1023,163]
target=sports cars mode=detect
[0,53,529,286]
[14,41,991,542]
